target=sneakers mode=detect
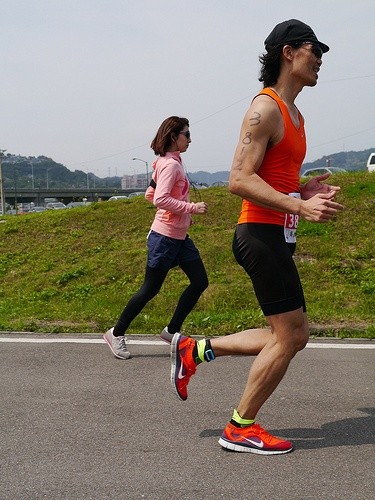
[218,420,294,455]
[171,332,197,401]
[159,326,174,345]
[103,327,131,360]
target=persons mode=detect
[101,116,209,360]
[170,18,346,457]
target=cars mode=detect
[0,201,84,215]
[301,167,347,178]
[128,192,144,198]
[211,181,229,187]
[108,196,127,202]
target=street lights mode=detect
[132,158,148,189]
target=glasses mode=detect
[180,132,190,139]
[302,41,323,59]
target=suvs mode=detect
[366,153,375,174]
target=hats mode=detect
[263,19,330,53]
